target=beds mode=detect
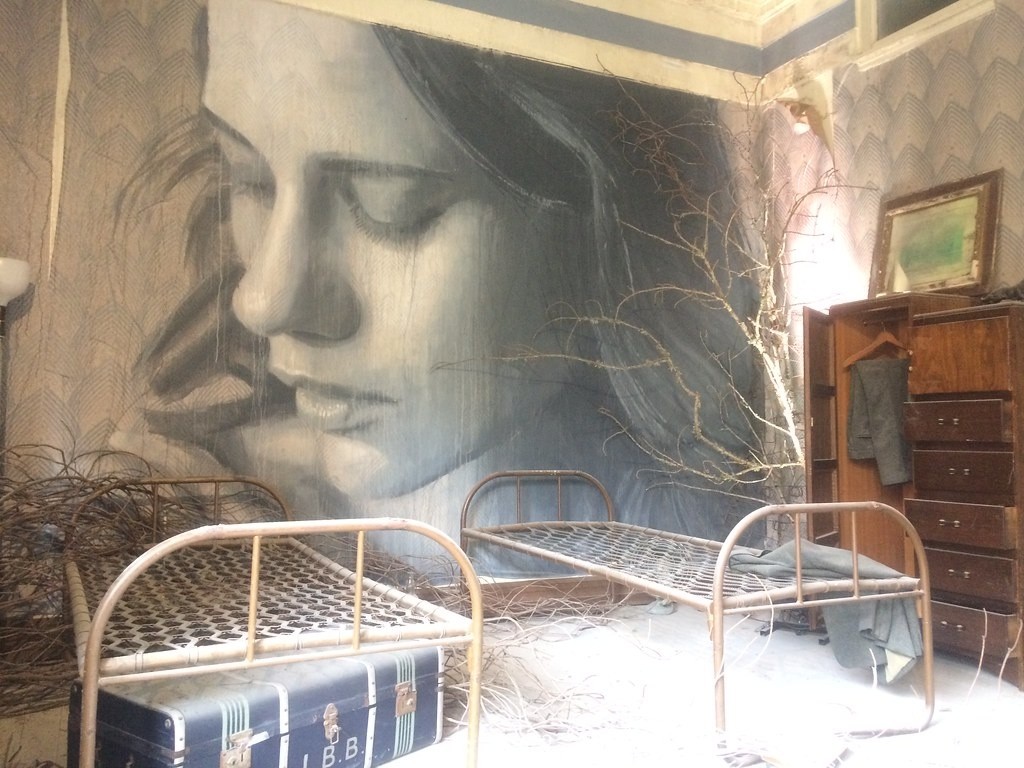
[458,470,935,751]
[57,477,484,768]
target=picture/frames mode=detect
[867,166,1001,299]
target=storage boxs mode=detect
[66,639,446,768]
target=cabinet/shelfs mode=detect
[802,291,1024,693]
